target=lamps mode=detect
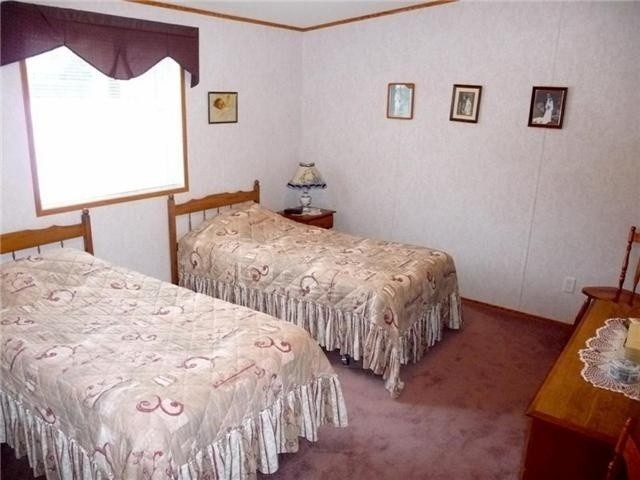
[287,161,329,213]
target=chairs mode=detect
[601,415,640,480]
[571,226,640,340]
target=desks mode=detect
[517,296,640,478]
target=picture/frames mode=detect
[386,83,415,118]
[207,91,238,125]
[526,86,570,129]
[449,83,484,124]
